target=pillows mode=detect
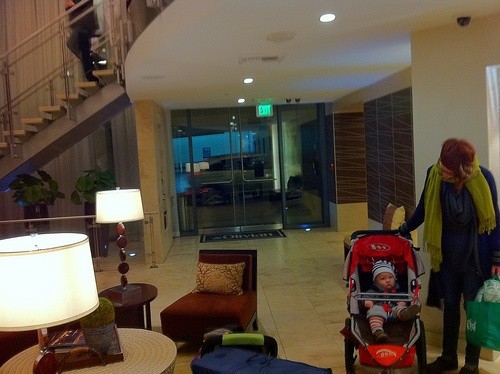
[383,203,405,230]
[193,262,245,296]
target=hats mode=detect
[372,260,398,283]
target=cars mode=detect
[188,153,274,207]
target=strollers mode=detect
[339,228,429,374]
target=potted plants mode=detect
[71,166,117,259]
[9,170,65,234]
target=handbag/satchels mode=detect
[465,276,500,351]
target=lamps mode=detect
[0,229,100,374]
[96,187,145,302]
[185,162,209,173]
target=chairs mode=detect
[160,250,258,341]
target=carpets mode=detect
[200,229,287,243]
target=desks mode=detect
[0,328,178,374]
[99,283,158,331]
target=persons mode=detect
[363,260,420,342]
[65,0,106,81]
[399,138,500,374]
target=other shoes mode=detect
[427,356,458,374]
[373,330,388,342]
[400,304,422,321]
[459,362,480,374]
